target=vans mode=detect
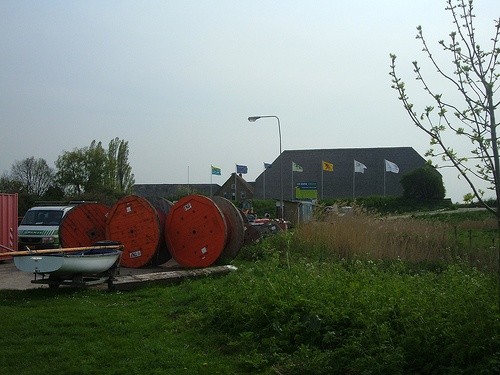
[17,205,74,251]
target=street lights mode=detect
[247,115,284,218]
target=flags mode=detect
[212,166,221,175]
[355,160,367,173]
[386,160,399,173]
[264,163,271,168]
[236,165,247,173]
[293,162,303,171]
[323,161,333,171]
